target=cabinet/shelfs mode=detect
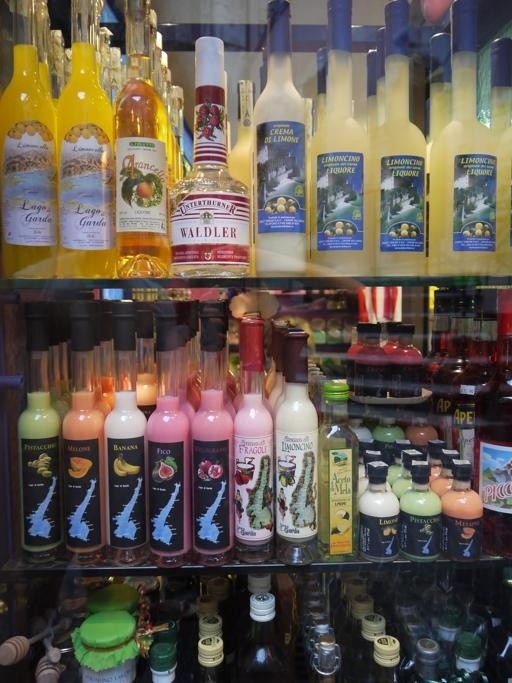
[0,275,512,578]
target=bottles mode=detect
[2,291,512,562]
[0,1,512,279]
[3,568,512,683]
